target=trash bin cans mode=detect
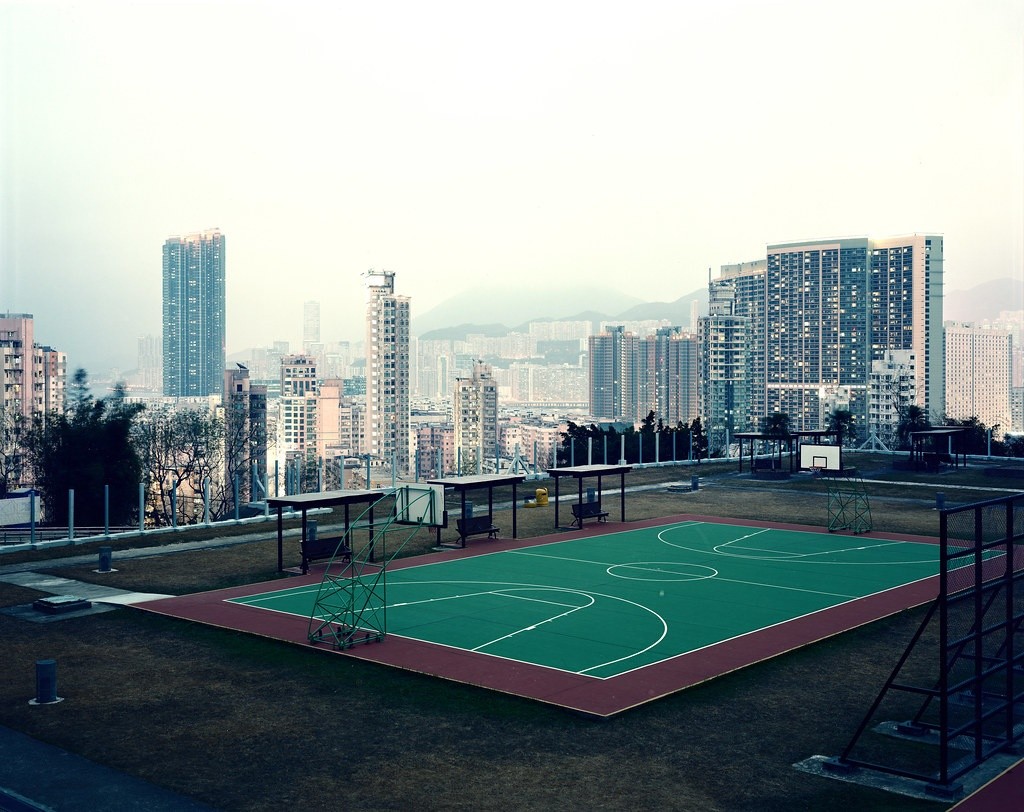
[535,488,549,507]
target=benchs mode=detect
[455,514,501,544]
[570,501,610,527]
[300,534,353,570]
